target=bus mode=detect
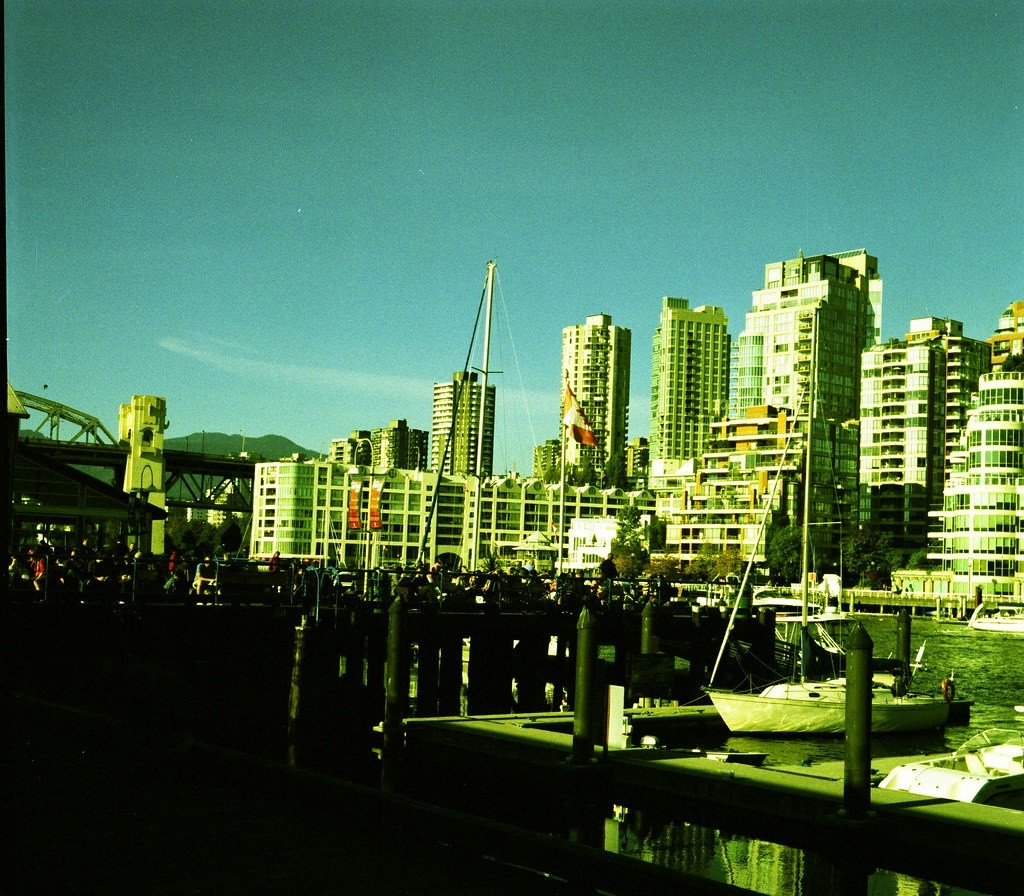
[195,556,306,607]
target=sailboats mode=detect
[698,298,977,738]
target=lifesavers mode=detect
[943,679,955,702]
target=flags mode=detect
[562,384,599,446]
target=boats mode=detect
[969,612,1024,633]
[670,748,771,767]
[878,703,1023,810]
[750,585,856,627]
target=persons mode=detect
[220,552,232,566]
[27,547,45,590]
[601,553,617,578]
[371,560,602,605]
[54,534,143,580]
[268,552,281,571]
[192,555,216,594]
[169,550,178,570]
[8,555,18,584]
